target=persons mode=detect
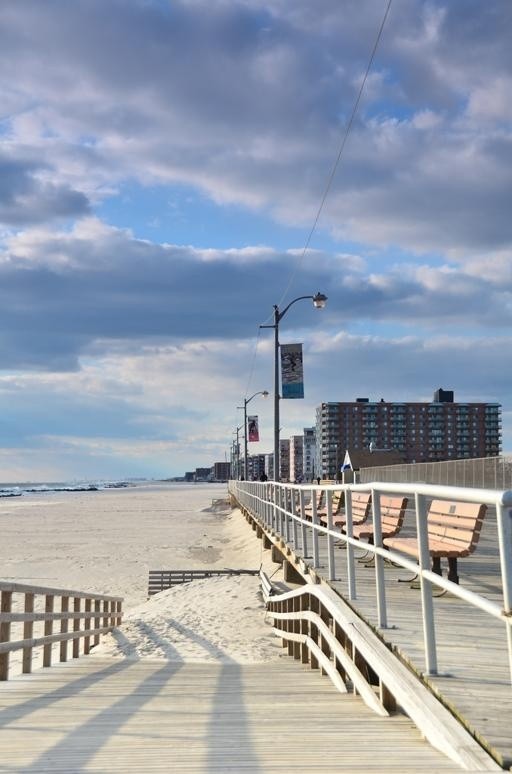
[260,471,267,482]
[317,476,321,484]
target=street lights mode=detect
[260,291,328,482]
[232,390,269,481]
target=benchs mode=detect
[296,489,487,597]
